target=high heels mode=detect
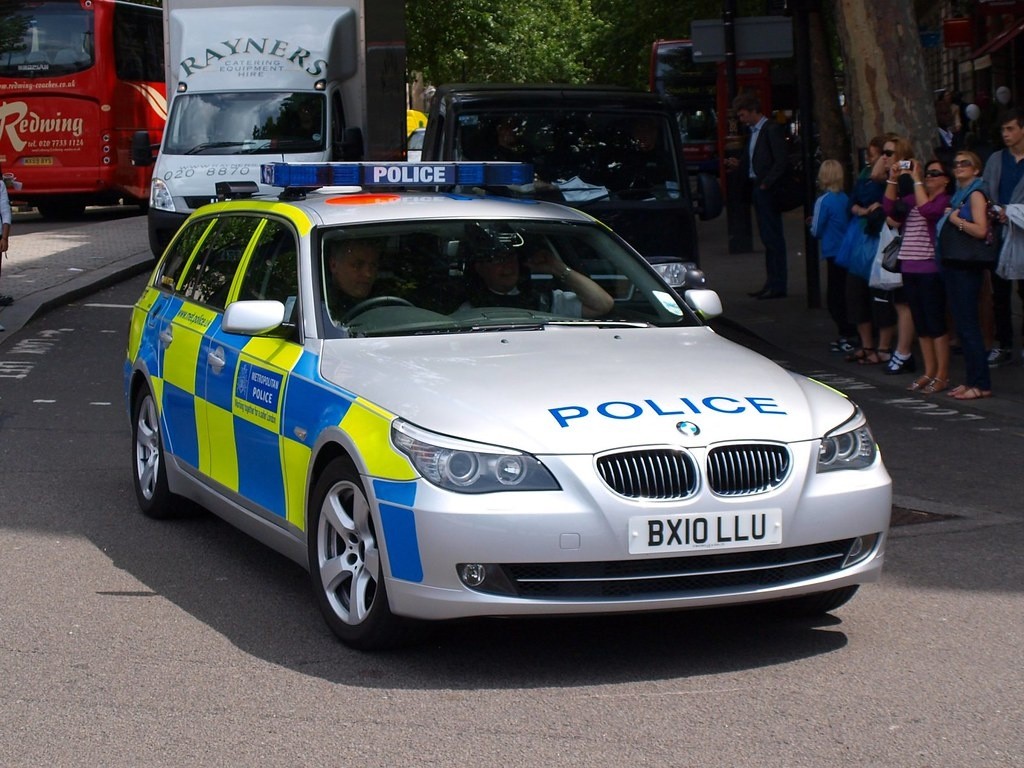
[885,353,916,374]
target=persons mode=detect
[494,123,523,161]
[0,180,14,305]
[805,91,1024,400]
[272,101,319,148]
[326,239,419,321]
[619,123,673,186]
[431,236,614,318]
[728,99,788,300]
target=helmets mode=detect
[463,222,518,262]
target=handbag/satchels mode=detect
[882,234,901,274]
[939,189,1000,261]
[868,222,904,290]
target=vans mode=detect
[650,38,773,173]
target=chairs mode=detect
[204,110,236,134]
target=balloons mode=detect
[965,104,980,120]
[996,86,1011,105]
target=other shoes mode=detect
[750,286,787,299]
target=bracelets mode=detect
[914,182,923,185]
[886,180,897,184]
[959,219,966,231]
[561,268,570,282]
[2,237,7,240]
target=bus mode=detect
[0,0,163,220]
[421,83,729,332]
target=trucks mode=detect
[130,0,413,262]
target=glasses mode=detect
[924,169,947,178]
[879,150,898,158]
[488,250,515,263]
[952,160,975,169]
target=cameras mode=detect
[897,160,912,170]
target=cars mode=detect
[124,161,896,652]
[407,129,428,162]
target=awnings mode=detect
[974,17,1024,57]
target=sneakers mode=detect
[987,347,1015,368]
[828,337,862,352]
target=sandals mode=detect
[954,387,993,400]
[905,375,936,392]
[947,383,970,397]
[845,346,875,363]
[857,348,894,366]
[919,377,951,394]
